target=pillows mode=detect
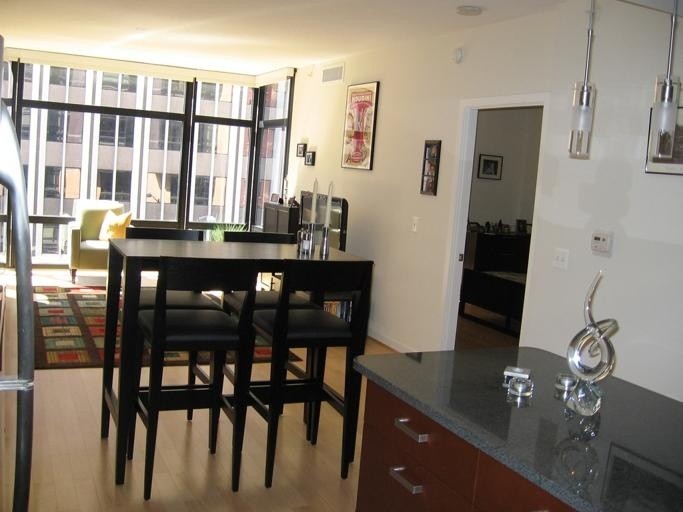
[99,210,132,240]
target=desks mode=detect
[351,377,683,512]
[101,239,374,485]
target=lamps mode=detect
[568,0,596,160]
[650,1,681,159]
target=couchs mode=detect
[68,204,126,285]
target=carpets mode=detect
[33,286,303,369]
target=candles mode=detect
[325,181,333,227]
[311,179,318,224]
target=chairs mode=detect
[223,231,323,442]
[255,260,374,489]
[125,226,223,447]
[127,256,259,500]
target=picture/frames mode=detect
[341,81,379,169]
[644,106,683,175]
[296,144,307,157]
[305,152,315,166]
[420,140,441,196]
[477,154,503,180]
